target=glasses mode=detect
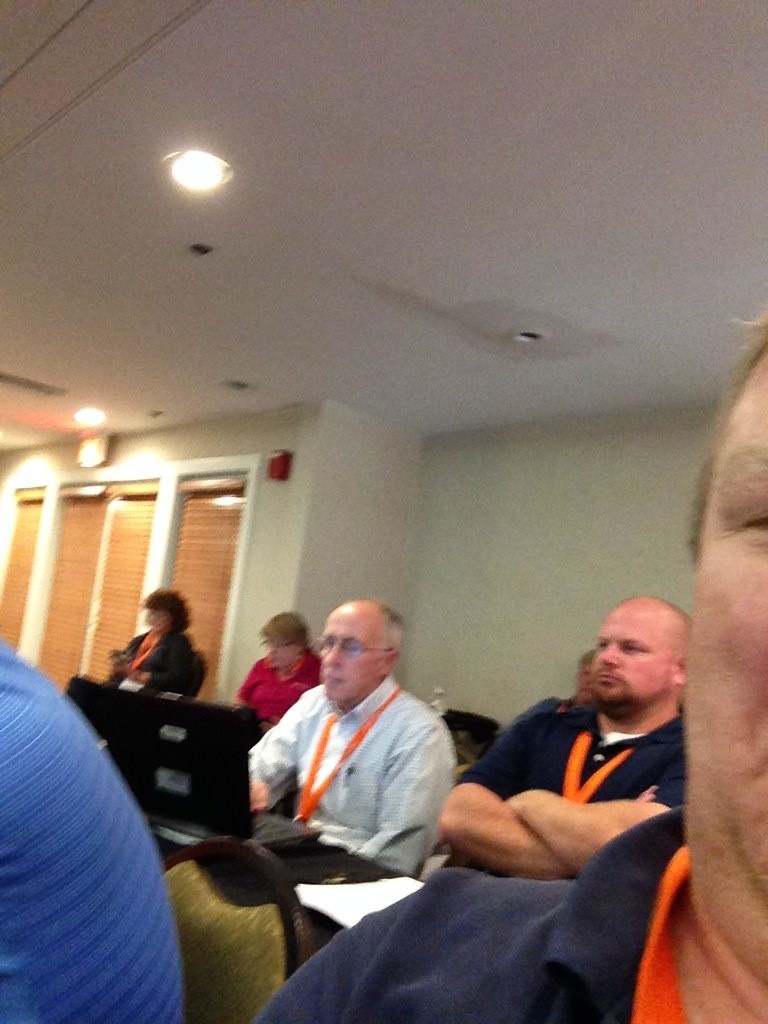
[264,640,298,646]
[308,638,394,655]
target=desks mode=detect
[258,807,431,967]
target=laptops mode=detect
[67,677,321,854]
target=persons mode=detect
[108,587,208,699]
[233,609,325,735]
[246,314,767,1024]
[0,639,186,1023]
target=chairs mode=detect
[150,831,311,1024]
[433,705,500,787]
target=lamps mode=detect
[172,149,228,191]
